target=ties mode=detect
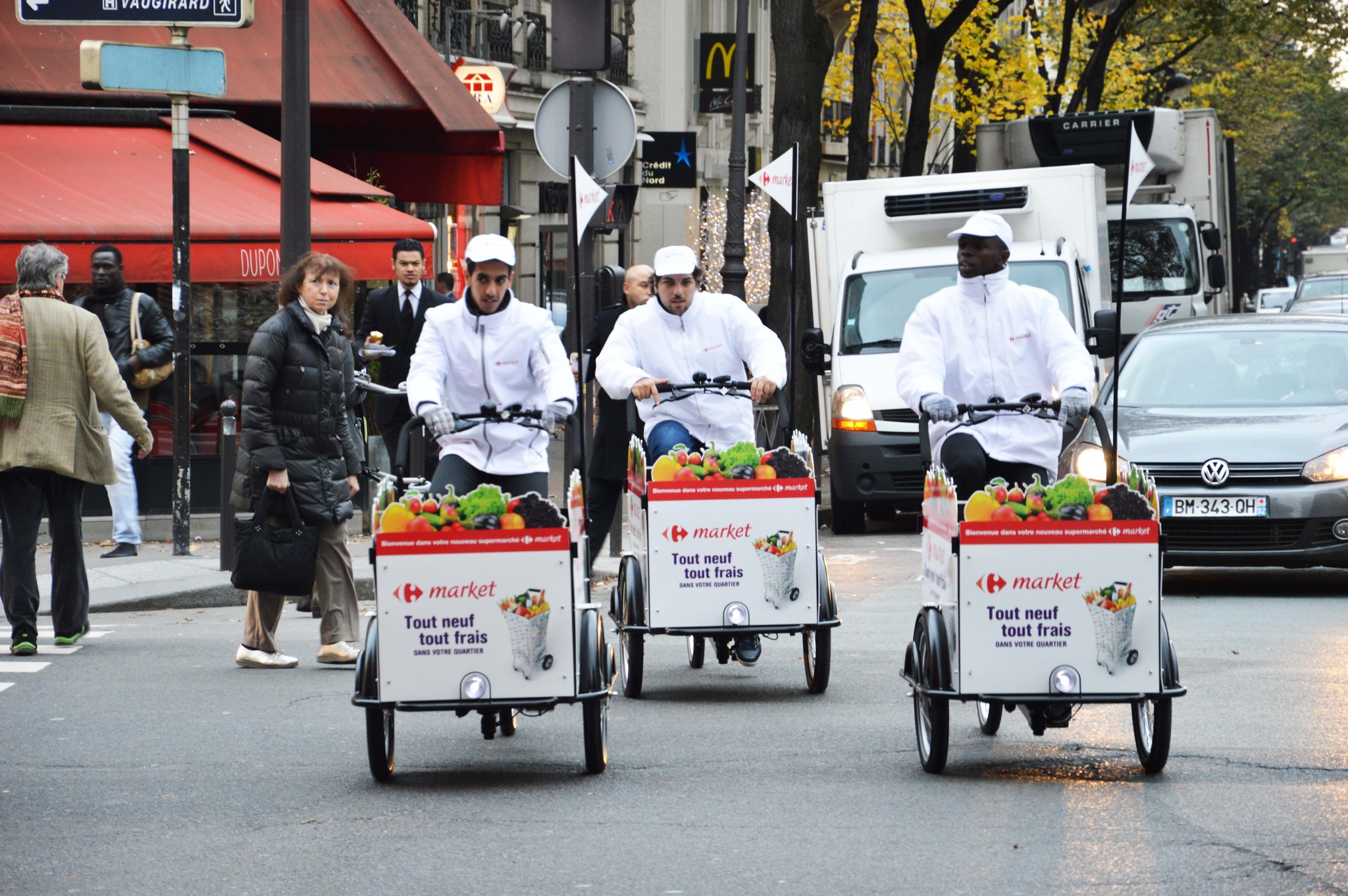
[399,290,414,338]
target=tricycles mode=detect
[894,391,1188,778]
[606,371,842,698]
[351,401,612,783]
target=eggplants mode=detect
[1024,474,1087,521]
[437,483,501,530]
[701,441,755,479]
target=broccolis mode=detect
[460,483,507,518]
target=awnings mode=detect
[1,125,436,285]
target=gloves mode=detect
[921,394,964,424]
[418,402,454,436]
[1057,388,1091,426]
[542,400,573,434]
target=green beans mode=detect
[674,450,726,481]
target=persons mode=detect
[586,263,656,569]
[227,251,365,670]
[0,239,154,655]
[407,233,578,722]
[898,211,1098,724]
[71,242,174,558]
[434,272,458,302]
[597,245,788,666]
[357,240,459,502]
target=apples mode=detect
[499,499,525,530]
[754,453,776,479]
[1087,490,1113,521]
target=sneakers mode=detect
[734,633,761,666]
[316,640,360,665]
[235,643,298,668]
[54,623,90,645]
[10,633,37,654]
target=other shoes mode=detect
[100,543,137,558]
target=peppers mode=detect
[650,449,683,481]
[964,484,1001,521]
[379,499,413,532]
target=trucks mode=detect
[969,100,1238,353]
[802,160,1126,534]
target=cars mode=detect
[1078,243,1348,591]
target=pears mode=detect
[403,498,465,532]
[991,483,1052,522]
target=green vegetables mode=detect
[722,442,761,471]
[1047,474,1095,509]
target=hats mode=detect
[948,212,1013,253]
[463,233,515,265]
[653,245,697,276]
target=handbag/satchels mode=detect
[131,292,174,388]
[230,486,320,596]
